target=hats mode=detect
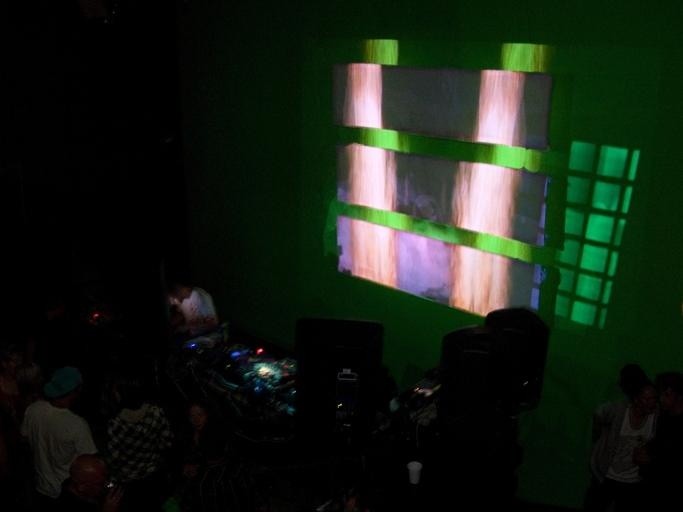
[43,367,83,398]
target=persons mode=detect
[314,461,378,512]
[584,362,661,512]
[634,370,682,512]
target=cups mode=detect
[406,461,423,486]
